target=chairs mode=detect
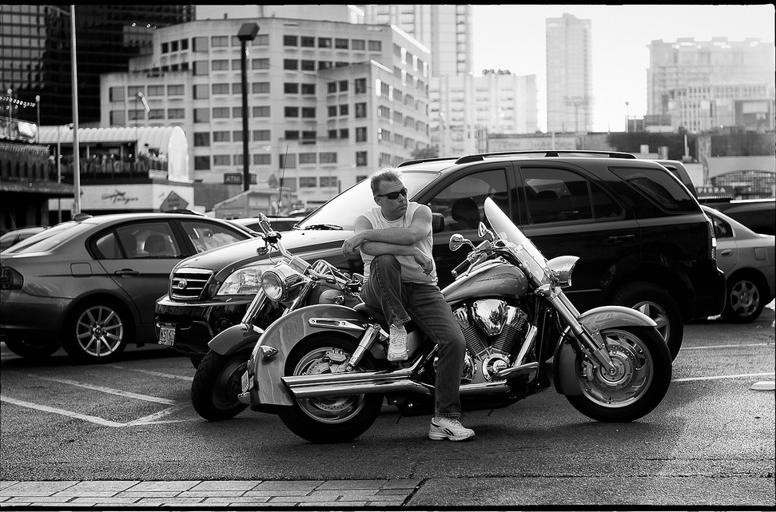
[99,233,175,257]
[434,189,561,232]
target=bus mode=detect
[38,125,194,213]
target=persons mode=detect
[341,170,475,441]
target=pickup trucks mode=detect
[655,160,776,231]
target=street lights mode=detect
[35,95,40,127]
[7,88,13,142]
[237,22,260,192]
[138,92,151,121]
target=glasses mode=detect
[376,187,408,199]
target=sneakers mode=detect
[387,324,409,362]
[429,416,475,442]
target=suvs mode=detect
[159,149,726,369]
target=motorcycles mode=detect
[184,213,372,422]
[239,196,673,446]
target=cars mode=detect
[0,211,266,366]
[1,227,54,253]
[698,206,776,324]
